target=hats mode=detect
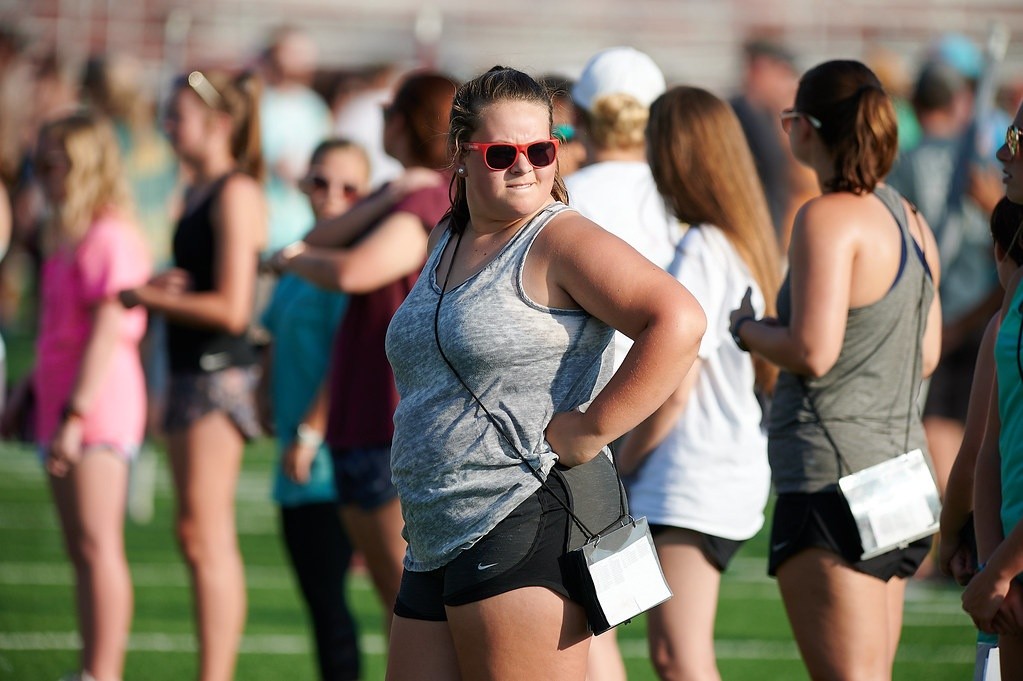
[569,46,669,112]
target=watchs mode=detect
[731,317,752,351]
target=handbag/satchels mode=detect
[838,448,940,560]
[559,516,673,635]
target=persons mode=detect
[0,28,1022,576]
[34,109,151,681]
[615,85,789,681]
[730,59,941,681]
[119,65,271,680]
[259,138,373,681]
[386,65,708,681]
[939,99,1022,681]
[272,74,459,680]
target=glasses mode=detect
[781,107,823,132]
[461,134,562,174]
[1005,123,1017,154]
[177,71,222,108]
[301,178,360,202]
